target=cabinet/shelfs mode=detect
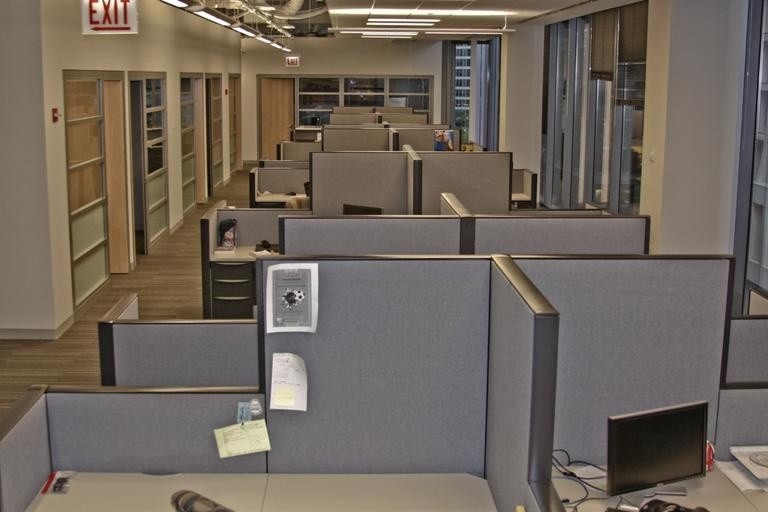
[212,261,255,320]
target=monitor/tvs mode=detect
[606,400,709,512]
[343,203,384,215]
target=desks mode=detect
[30,471,500,510]
[250,125,539,216]
[551,460,767,510]
[209,246,280,262]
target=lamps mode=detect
[159,0,296,53]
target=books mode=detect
[214,246,236,256]
[716,446,768,493]
